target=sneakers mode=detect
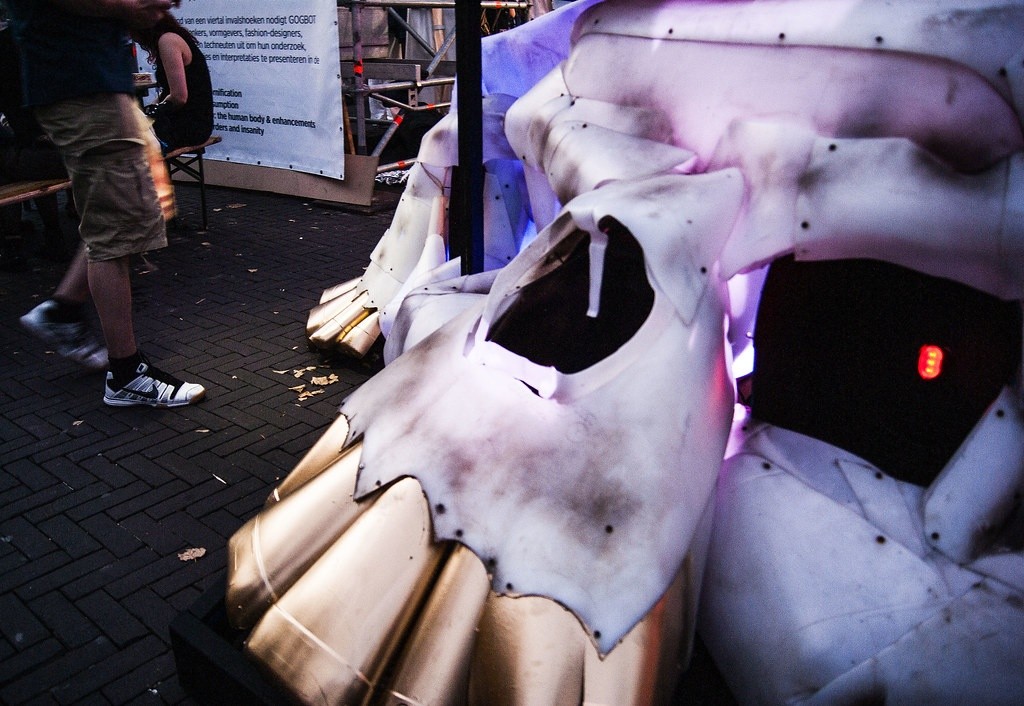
[105,364,204,407]
[20,302,109,369]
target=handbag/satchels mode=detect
[143,99,172,121]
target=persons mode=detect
[134,12,214,155]
[9,0,205,409]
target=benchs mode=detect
[0,136,224,232]
